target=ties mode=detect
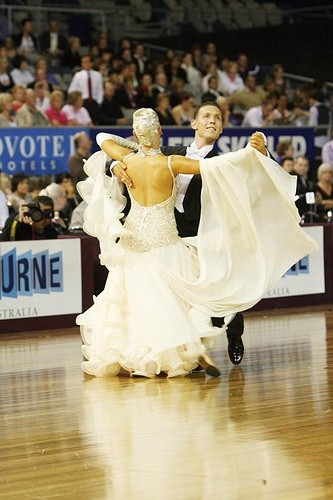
[87,72,92,104]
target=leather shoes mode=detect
[227,335,245,365]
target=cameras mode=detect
[23,202,54,222]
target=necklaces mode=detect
[138,146,162,156]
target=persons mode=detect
[0,18,333,241]
[109,102,276,370]
[96,109,267,378]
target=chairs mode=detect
[0,0,295,60]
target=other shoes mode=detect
[198,354,221,377]
